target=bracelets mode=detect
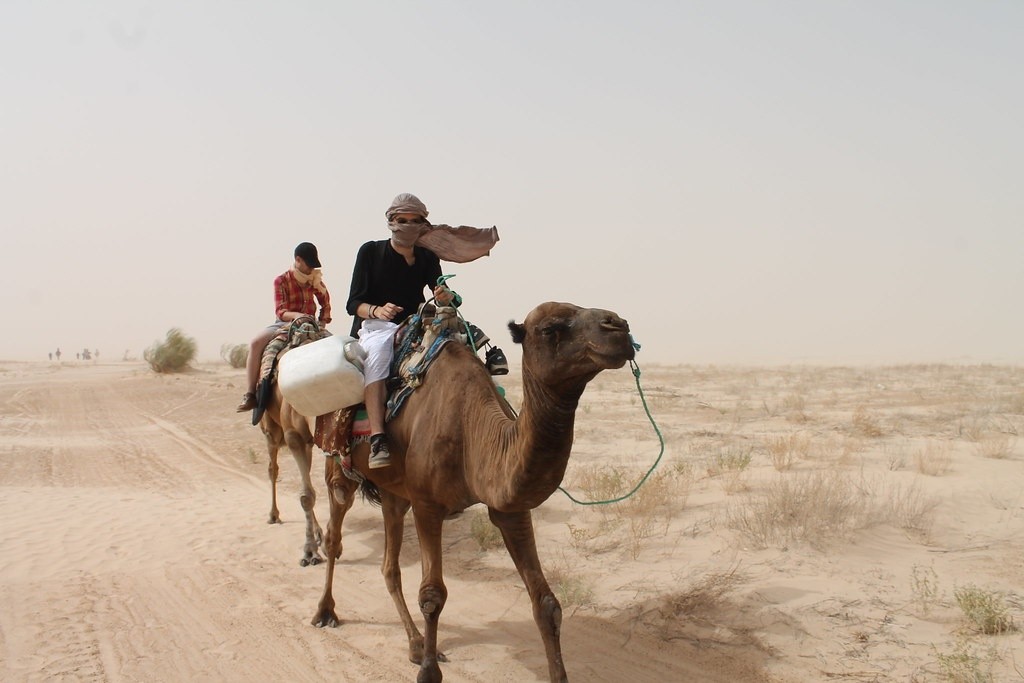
[368,304,375,319]
[372,306,380,319]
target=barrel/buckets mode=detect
[277,334,368,418]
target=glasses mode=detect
[394,217,421,224]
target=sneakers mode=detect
[484,342,509,376]
[368,438,392,469]
[467,325,490,351]
[237,393,258,412]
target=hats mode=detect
[295,243,321,268]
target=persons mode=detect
[236,242,331,414]
[348,193,500,469]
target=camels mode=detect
[311,302,637,683]
[252,325,334,566]
